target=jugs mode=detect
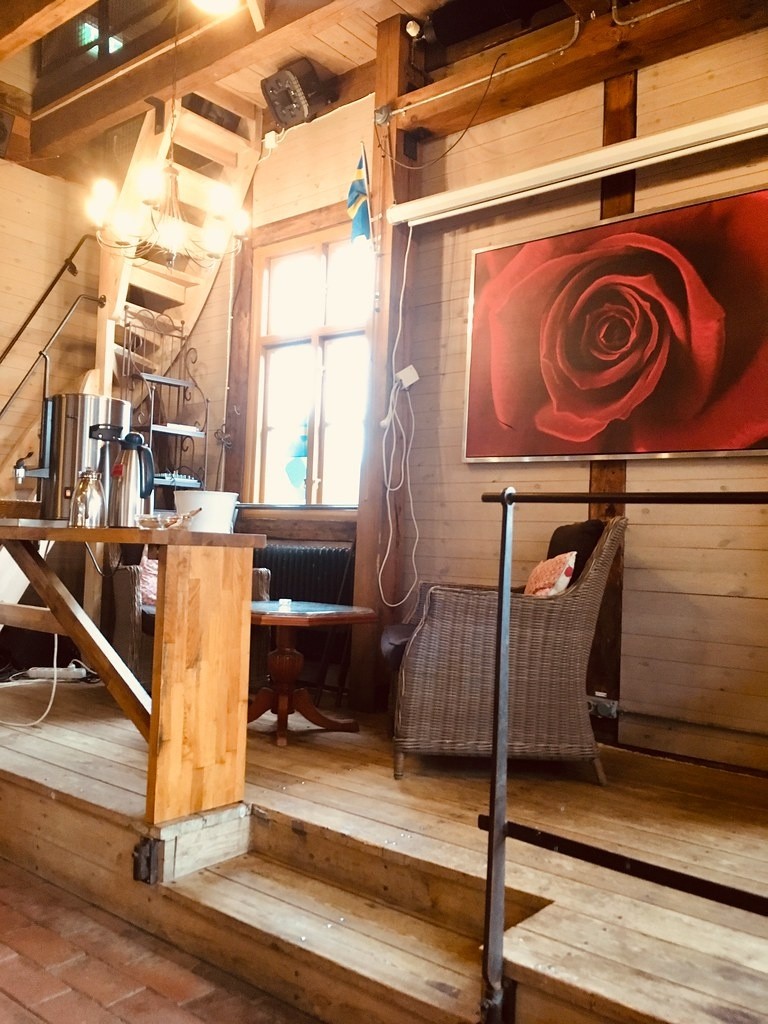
[68,468,106,529]
[109,432,154,527]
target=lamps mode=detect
[85,0,253,273]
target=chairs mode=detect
[391,511,627,788]
[103,541,271,685]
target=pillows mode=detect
[525,551,575,597]
[138,557,160,610]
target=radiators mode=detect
[253,545,355,665]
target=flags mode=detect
[348,155,370,242]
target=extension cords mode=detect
[27,667,86,679]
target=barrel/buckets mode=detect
[39,392,131,521]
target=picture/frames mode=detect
[462,183,767,462]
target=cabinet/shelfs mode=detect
[122,305,209,519]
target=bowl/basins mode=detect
[174,490,239,534]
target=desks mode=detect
[0,519,268,823]
[246,600,378,745]
